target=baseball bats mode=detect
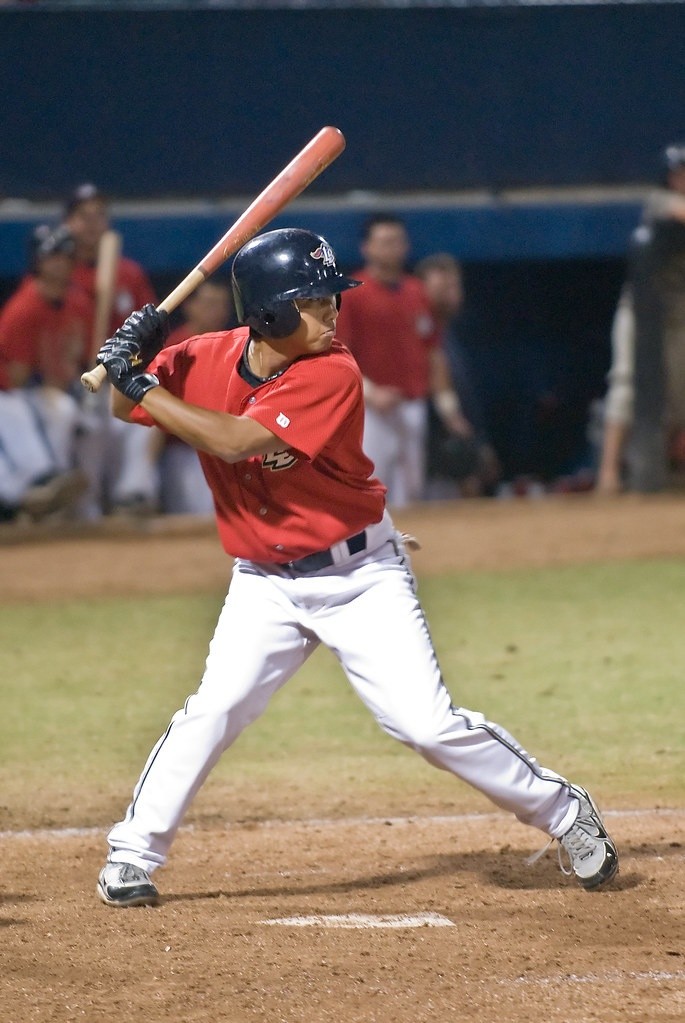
[81,125,346,393]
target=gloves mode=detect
[96,304,171,405]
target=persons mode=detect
[95,228,620,908]
[0,144,685,527]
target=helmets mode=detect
[65,184,104,212]
[658,145,685,168]
[29,224,72,262]
[232,227,361,338]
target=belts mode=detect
[279,530,367,573]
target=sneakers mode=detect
[527,785,619,892]
[96,862,159,906]
